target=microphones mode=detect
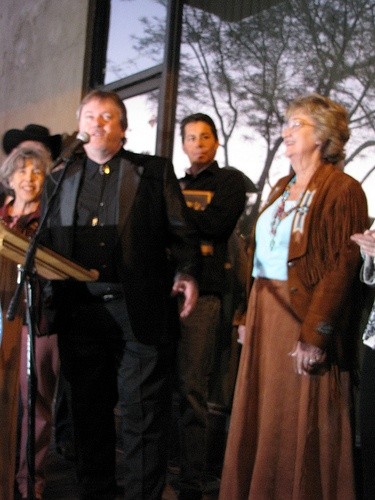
[53,132,90,168]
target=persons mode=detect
[0,86,375,500]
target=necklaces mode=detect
[269,175,304,233]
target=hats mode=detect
[3,125,65,161]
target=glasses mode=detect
[283,118,322,132]
